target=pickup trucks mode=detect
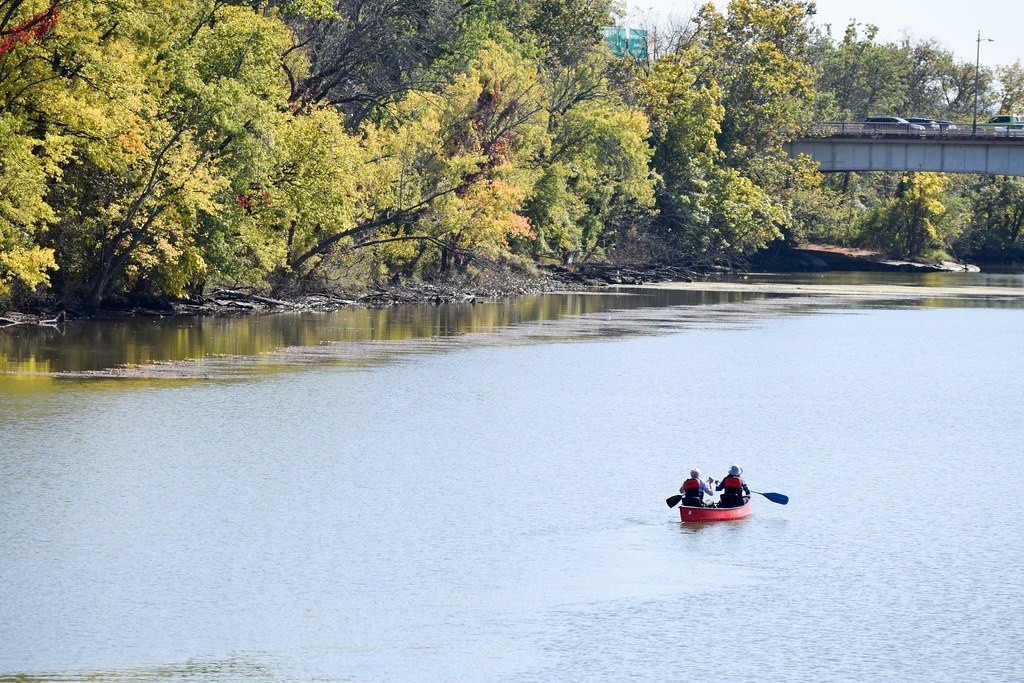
[975,116,1024,130]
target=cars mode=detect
[861,117,957,129]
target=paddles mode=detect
[666,479,712,508]
[721,482,789,505]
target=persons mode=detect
[715,464,750,507]
[679,468,714,507]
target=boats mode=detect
[678,496,751,524]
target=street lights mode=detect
[972,29,994,134]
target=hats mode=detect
[727,465,743,475]
[690,468,699,477]
